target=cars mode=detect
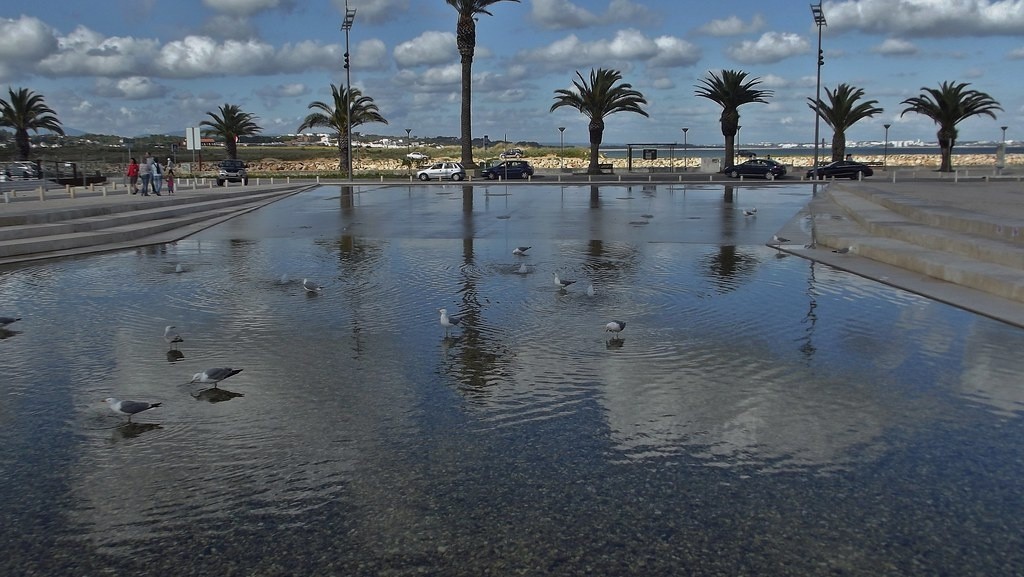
[6,161,44,179]
[735,150,757,159]
[883,123,891,169]
[500,149,524,160]
[407,152,430,160]
[723,158,788,180]
[806,160,875,183]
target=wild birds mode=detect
[0,316,23,341]
[832,248,850,255]
[773,234,791,248]
[552,272,577,290]
[190,366,244,390]
[303,278,325,296]
[101,396,164,424]
[605,319,626,338]
[743,208,757,218]
[176,264,185,273]
[439,309,462,341]
[163,326,183,351]
[512,246,533,257]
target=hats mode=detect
[167,158,170,160]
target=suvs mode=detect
[482,160,535,182]
[417,161,466,182]
[217,159,250,186]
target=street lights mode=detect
[1001,126,1008,145]
[810,0,827,177]
[406,128,411,154]
[737,126,741,163]
[682,127,689,170]
[340,0,359,182]
[558,126,566,168]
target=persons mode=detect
[144,152,155,194]
[752,154,757,164]
[139,158,150,196]
[126,158,139,194]
[152,159,163,196]
[166,158,175,176]
[165,172,175,195]
[846,154,853,161]
